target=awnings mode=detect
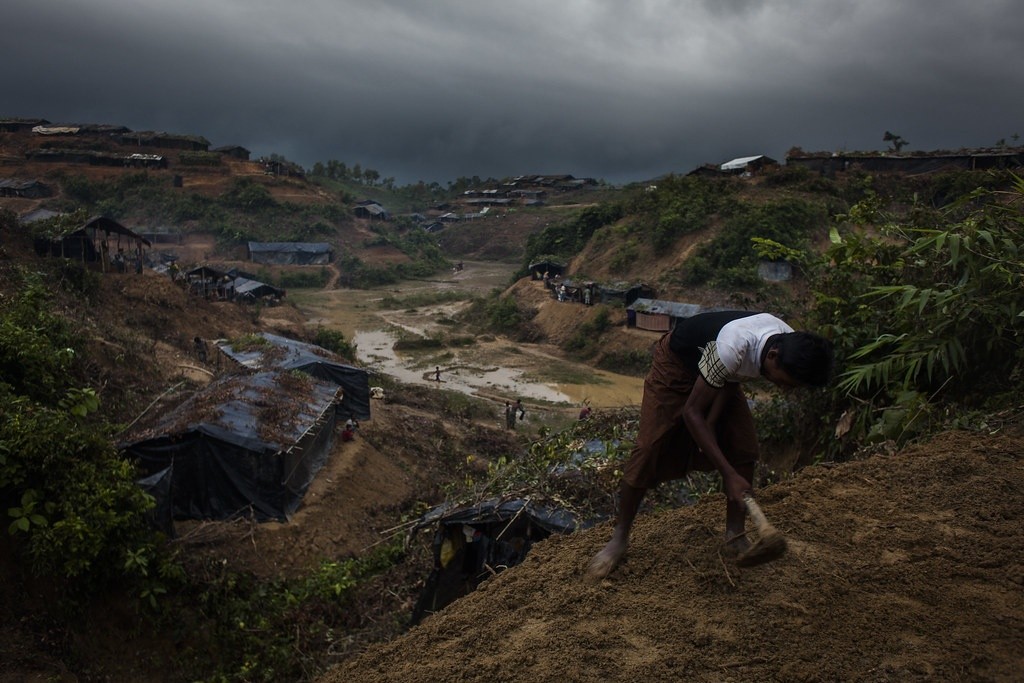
[184,265,285,301]
[354,172,601,233]
[58,213,150,259]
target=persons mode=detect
[99,239,144,274]
[580,405,592,420]
[588,311,838,581]
[193,336,209,362]
[433,365,441,381]
[342,412,361,440]
[506,398,525,430]
[544,271,592,306]
